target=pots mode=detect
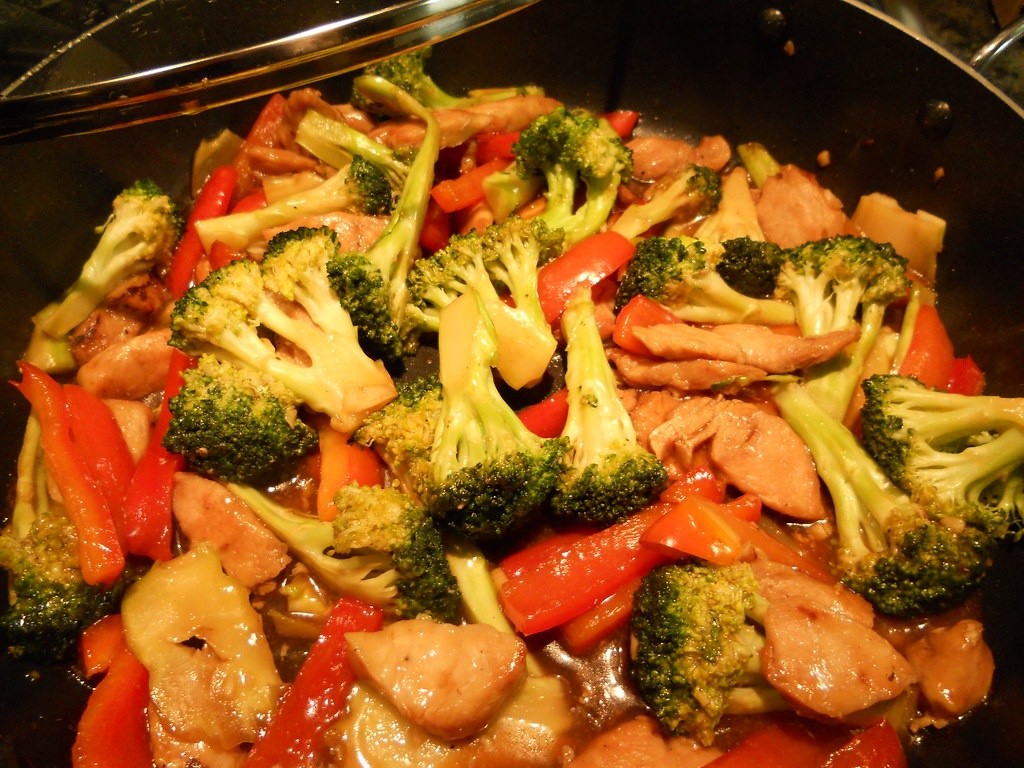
[0,0,1024,768]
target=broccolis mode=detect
[0,53,1023,768]
